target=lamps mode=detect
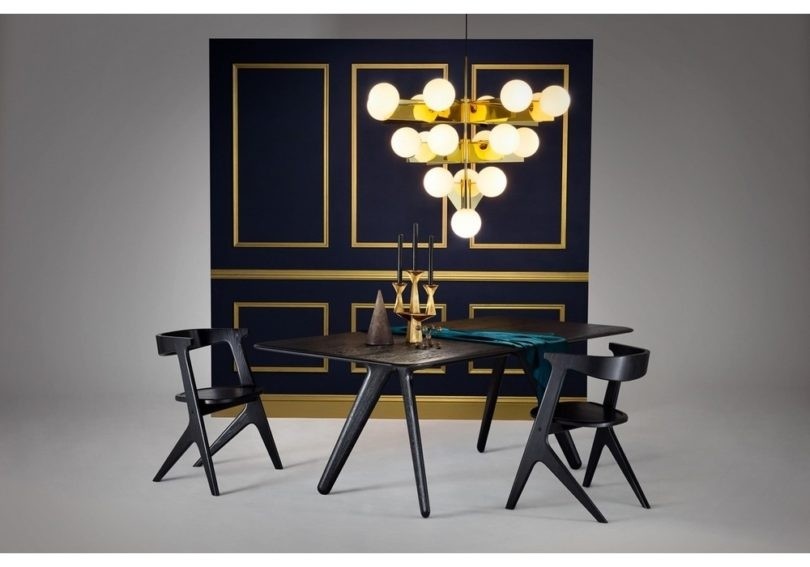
[367,14,570,239]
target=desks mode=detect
[255,315,633,516]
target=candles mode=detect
[427,235,434,285]
[412,223,418,271]
[398,234,402,286]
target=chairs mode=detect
[153,327,284,496]
[504,342,651,522]
